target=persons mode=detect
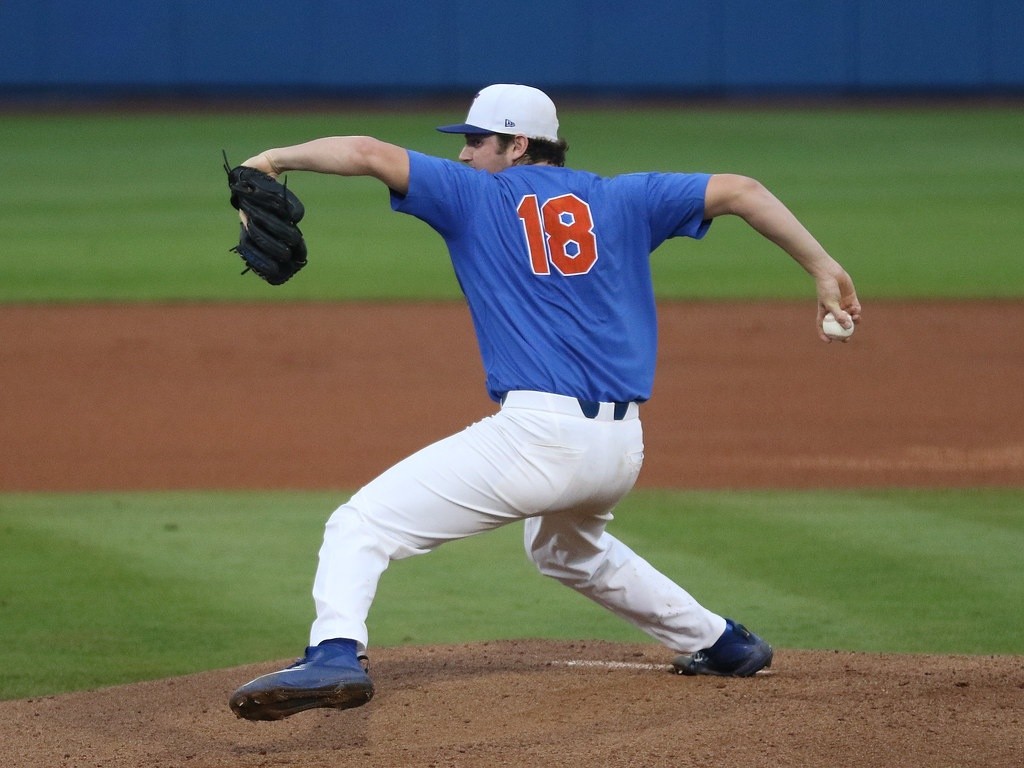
[223,83,862,721]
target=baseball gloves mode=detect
[228,166,307,286]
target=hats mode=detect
[436,84,559,143]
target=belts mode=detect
[578,399,629,420]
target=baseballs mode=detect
[822,310,854,339]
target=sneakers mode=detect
[672,617,773,676]
[229,638,375,722]
